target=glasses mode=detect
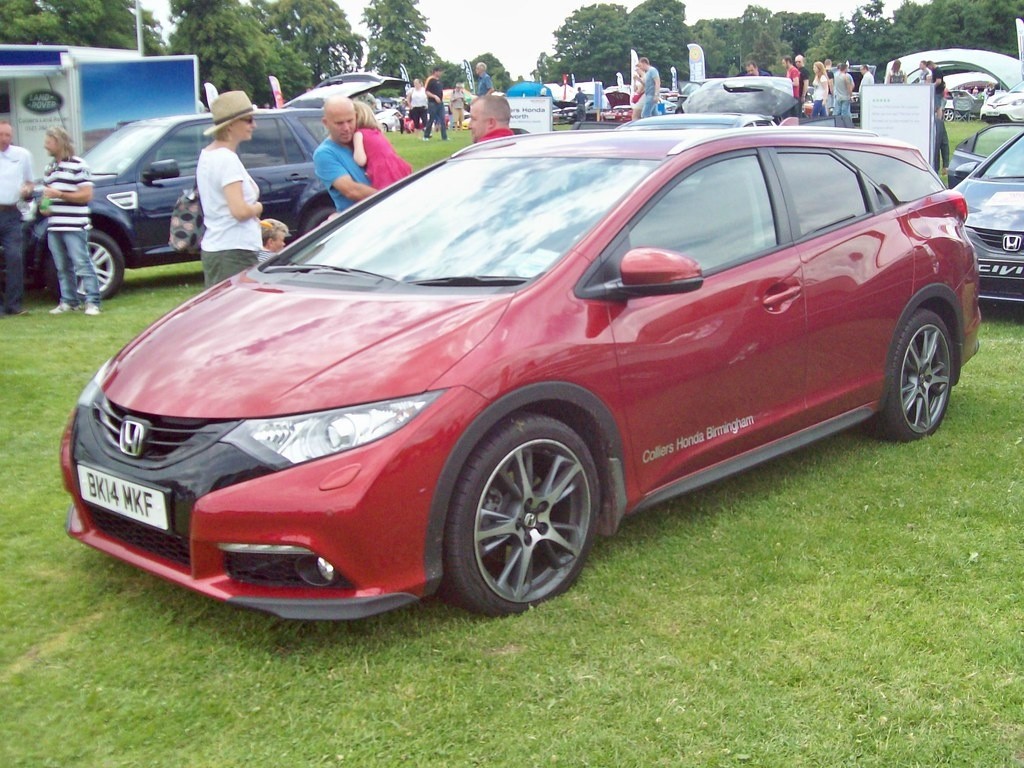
[795,61,801,63]
[240,118,253,124]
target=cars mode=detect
[58,125,983,623]
[615,112,780,131]
[980,79,1024,124]
[675,74,815,112]
[942,90,984,122]
[551,86,684,122]
[947,122,1024,305]
[831,62,877,121]
[0,71,412,306]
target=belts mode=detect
[0,205,16,210]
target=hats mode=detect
[203,90,259,135]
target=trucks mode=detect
[0,40,201,186]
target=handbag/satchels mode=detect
[169,189,206,255]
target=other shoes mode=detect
[423,138,429,141]
[6,308,31,316]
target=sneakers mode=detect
[49,303,81,313]
[84,306,103,315]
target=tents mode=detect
[885,48,1023,92]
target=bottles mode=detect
[40,197,50,211]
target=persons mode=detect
[197,93,277,111]
[313,91,415,213]
[781,54,855,128]
[573,87,588,122]
[952,82,995,100]
[258,218,289,266]
[631,57,660,120]
[886,60,907,84]
[422,66,450,142]
[858,64,874,94]
[195,90,263,289]
[0,119,35,316]
[450,82,465,132]
[37,126,100,316]
[475,62,495,96]
[406,78,432,140]
[919,60,950,176]
[467,95,514,143]
[736,52,773,77]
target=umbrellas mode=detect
[280,72,414,108]
[682,75,799,117]
